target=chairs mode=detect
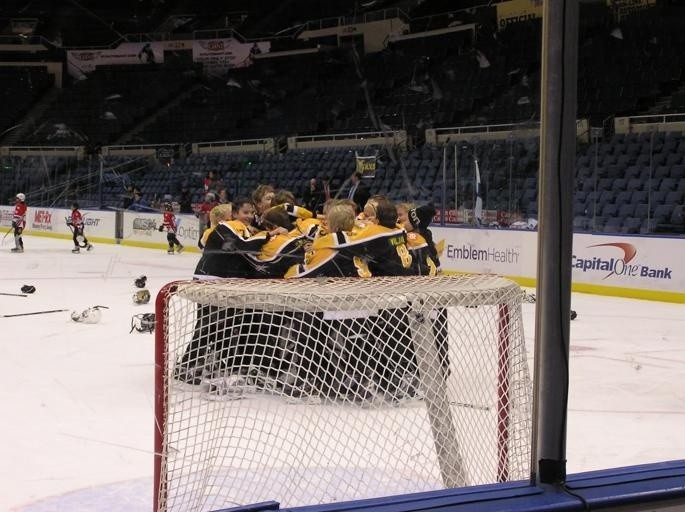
[0,0,685,234]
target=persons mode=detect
[158,201,183,252]
[175,168,452,404]
[64,202,92,253]
[9,191,28,253]
[119,181,174,214]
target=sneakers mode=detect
[168,245,183,252]
[170,361,452,400]
[72,244,92,251]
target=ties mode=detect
[348,185,356,199]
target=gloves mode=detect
[159,225,164,232]
[12,221,16,228]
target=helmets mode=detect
[16,193,26,202]
[137,289,151,304]
[141,313,155,332]
[84,309,101,324]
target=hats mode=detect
[408,203,436,229]
